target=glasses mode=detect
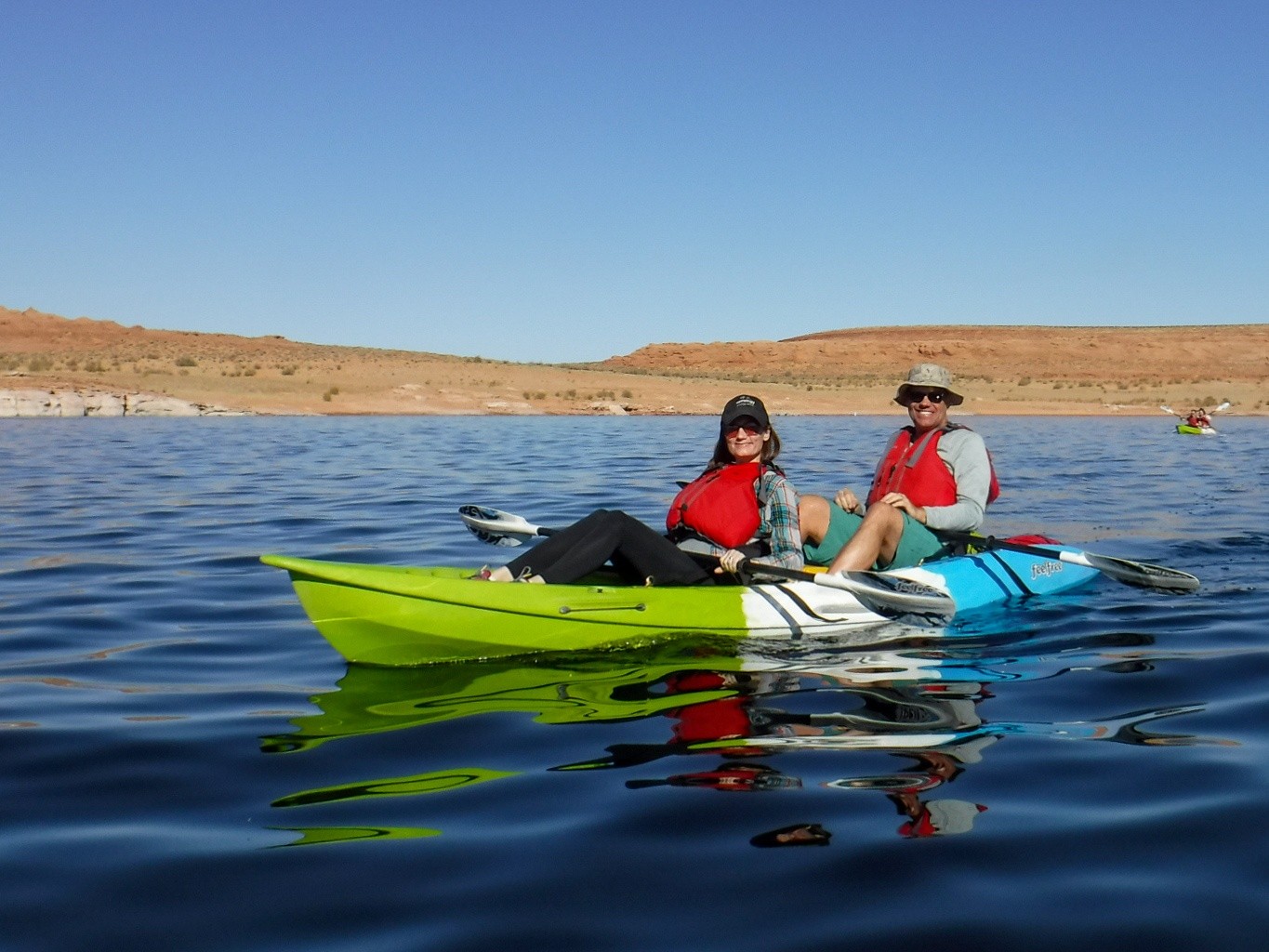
[723,422,767,437]
[906,391,947,404]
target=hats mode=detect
[721,395,768,427]
[893,363,964,409]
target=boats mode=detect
[1175,422,1217,435]
[259,531,1121,668]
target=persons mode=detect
[798,364,991,577]
[465,394,805,588]
[1181,409,1210,428]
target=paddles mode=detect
[458,503,957,629]
[1207,401,1230,416]
[1160,405,1223,436]
[676,479,1200,596]
